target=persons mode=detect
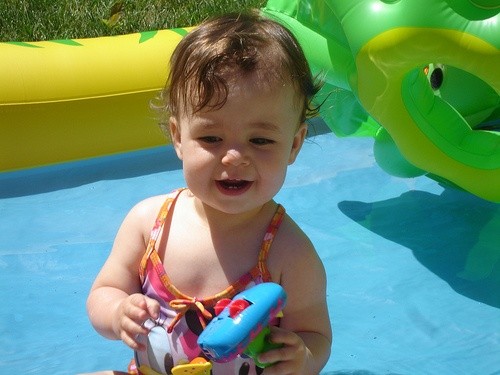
[86,12,339,375]
